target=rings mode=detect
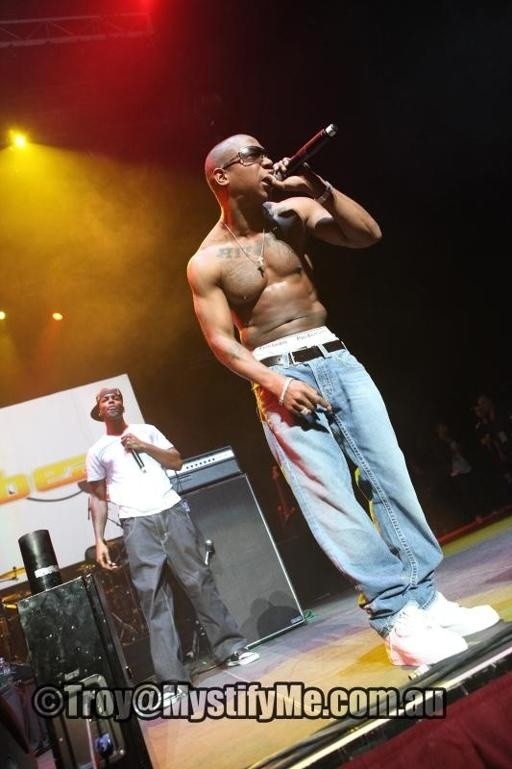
[301,407,310,416]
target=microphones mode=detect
[130,448,147,473]
[205,539,213,564]
[271,124,338,180]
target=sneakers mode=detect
[384,604,468,667]
[425,592,500,636]
[162,687,182,708]
[226,648,260,668]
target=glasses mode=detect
[220,146,272,171]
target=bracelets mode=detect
[278,376,293,406]
[315,180,333,206]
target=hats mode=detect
[91,387,123,422]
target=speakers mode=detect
[178,473,306,650]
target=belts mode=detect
[260,339,346,366]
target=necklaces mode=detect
[219,202,269,274]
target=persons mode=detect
[185,132,500,670]
[84,385,261,708]
